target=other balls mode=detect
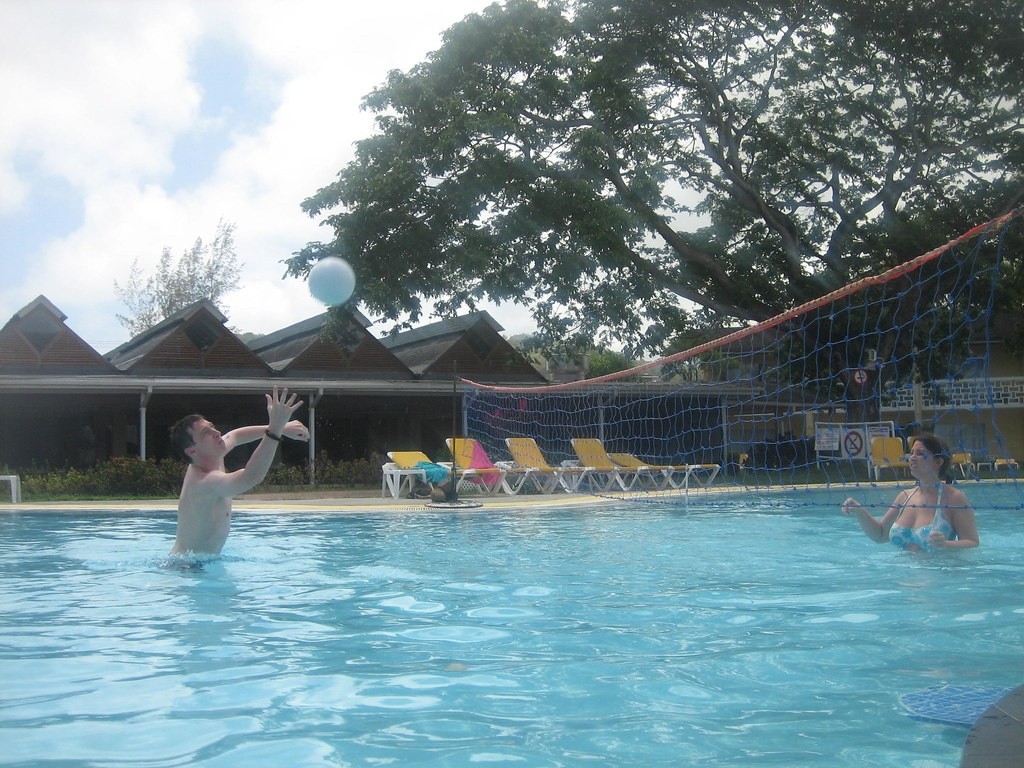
[307,257,356,306]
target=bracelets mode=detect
[263,430,282,441]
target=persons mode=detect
[169,384,312,555]
[841,434,980,550]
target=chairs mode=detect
[994,459,1019,471]
[871,436,971,481]
[382,438,720,500]
[0,475,21,504]
